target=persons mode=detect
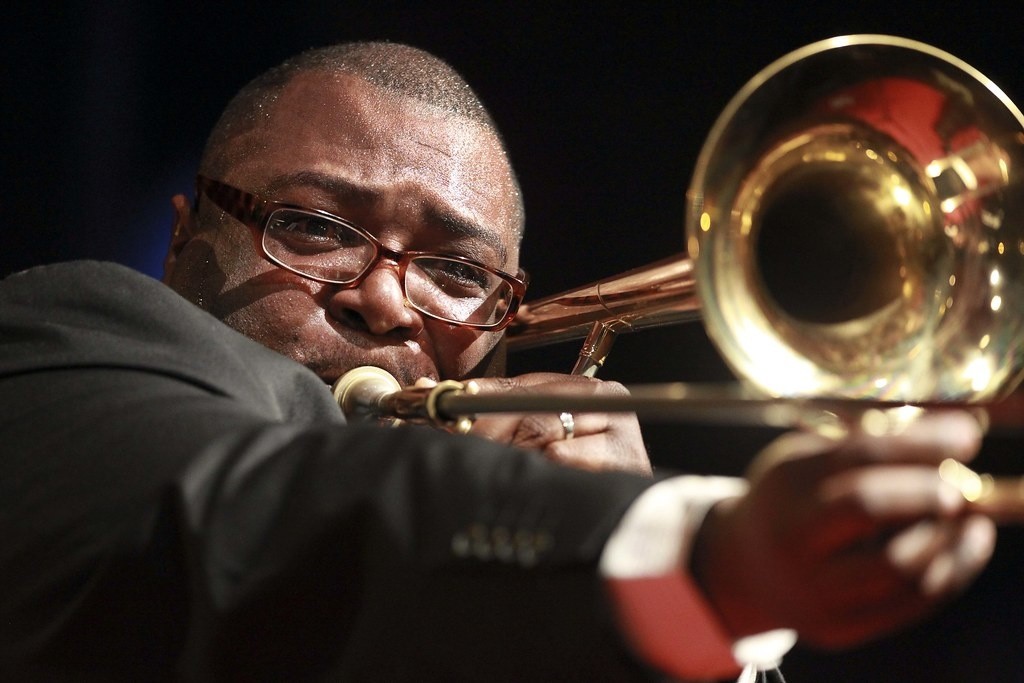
[0,36,998,683]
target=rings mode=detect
[558,410,576,440]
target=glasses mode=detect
[196,174,530,332]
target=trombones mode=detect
[325,32,1024,436]
